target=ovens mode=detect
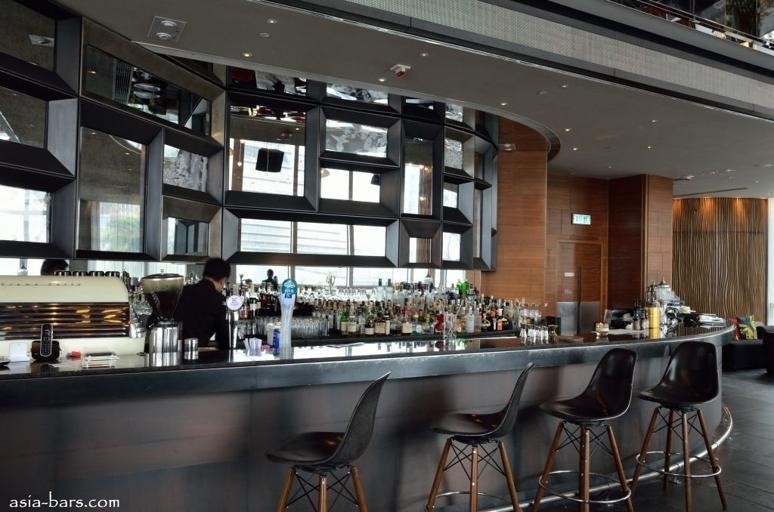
[1,270,150,361]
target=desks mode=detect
[0,308,736,512]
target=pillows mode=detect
[738,315,758,340]
[729,317,741,342]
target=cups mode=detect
[257,316,331,339]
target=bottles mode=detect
[641,310,646,330]
[645,311,650,329]
[633,309,640,330]
[519,323,549,346]
[224,278,542,334]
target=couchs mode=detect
[722,321,767,373]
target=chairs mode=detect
[426,361,536,512]
[638,341,727,512]
[267,372,392,512]
[539,348,637,512]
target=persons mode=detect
[173,257,231,352]
[40,259,69,278]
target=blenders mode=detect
[141,269,184,369]
